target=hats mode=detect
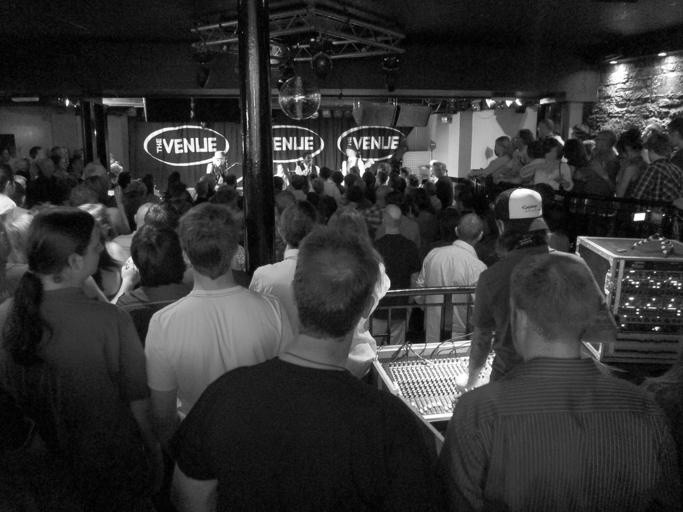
[495,187,548,233]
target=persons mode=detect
[0,119,683,384]
[439,253,681,512]
[144,201,295,445]
[0,209,166,512]
[162,227,437,512]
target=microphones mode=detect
[358,150,362,163]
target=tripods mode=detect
[629,228,677,256]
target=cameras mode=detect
[630,205,669,227]
